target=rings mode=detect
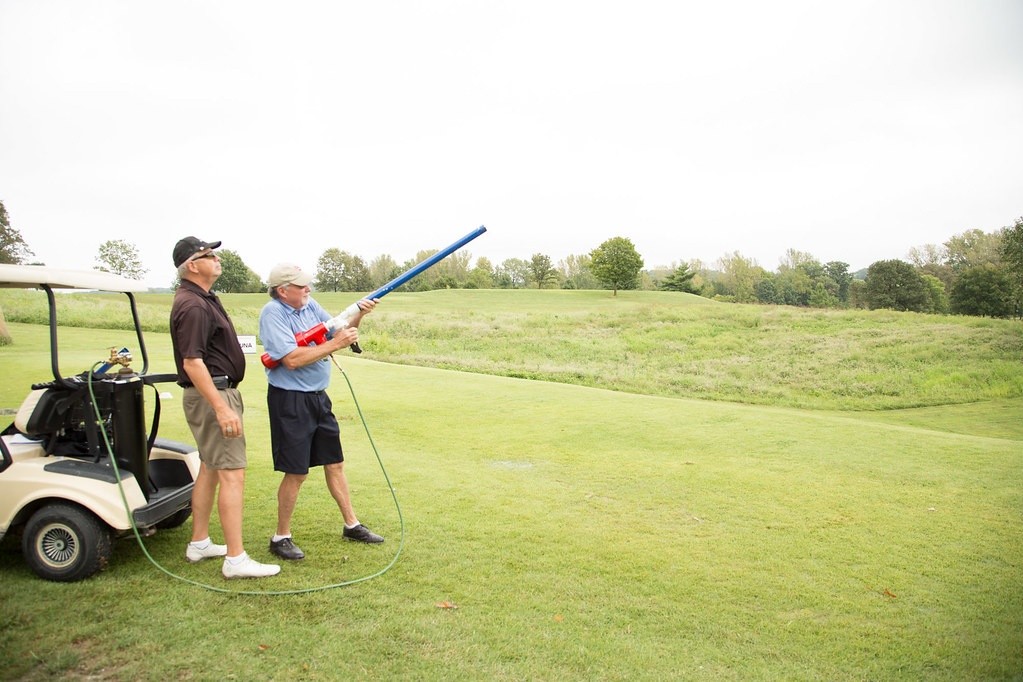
[227,426,232,432]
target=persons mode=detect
[170,237,280,578]
[259,263,384,559]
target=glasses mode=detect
[192,251,217,261]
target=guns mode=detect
[260,223,488,369]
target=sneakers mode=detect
[269,537,305,560]
[222,556,281,580]
[185,543,227,565]
[342,524,385,545]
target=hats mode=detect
[267,263,314,288]
[173,236,221,268]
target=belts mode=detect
[183,382,237,388]
